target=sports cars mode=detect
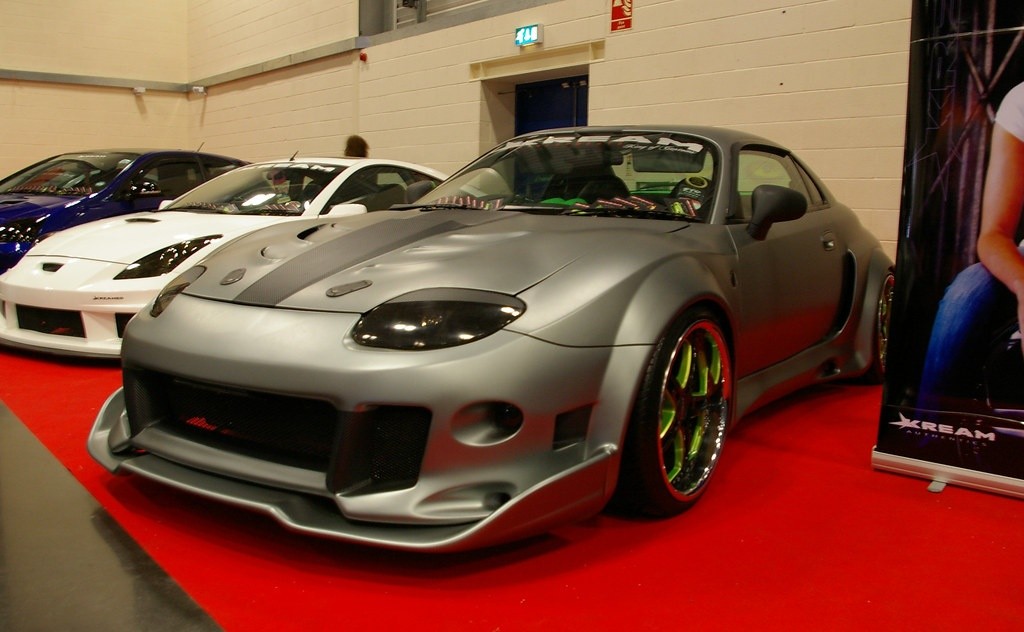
[1,146,294,273]
[0,154,488,362]
[88,125,899,555]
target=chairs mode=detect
[298,180,325,204]
[668,176,744,220]
[576,176,631,205]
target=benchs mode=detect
[341,183,407,212]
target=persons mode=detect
[344,135,378,184]
[914,83,1024,460]
